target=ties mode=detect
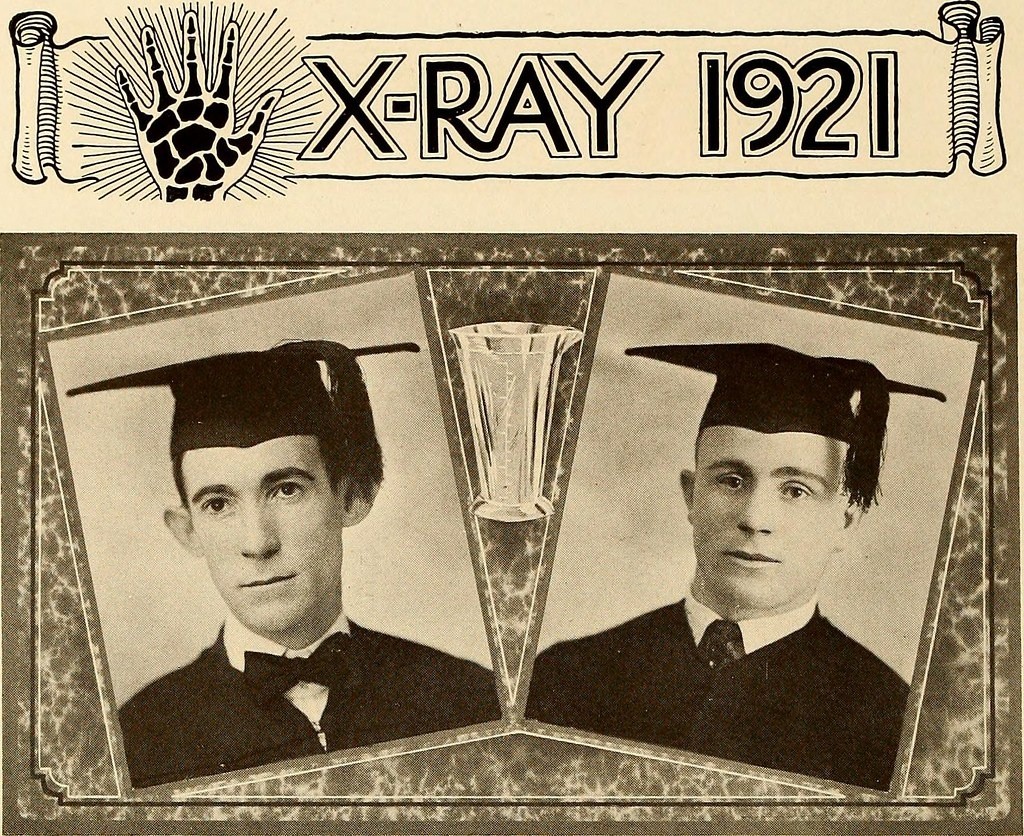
[697,620,746,671]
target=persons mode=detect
[523,343,948,794]
[61,338,505,791]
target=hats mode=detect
[623,343,947,515]
[66,342,422,492]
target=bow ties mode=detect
[243,632,354,707]
